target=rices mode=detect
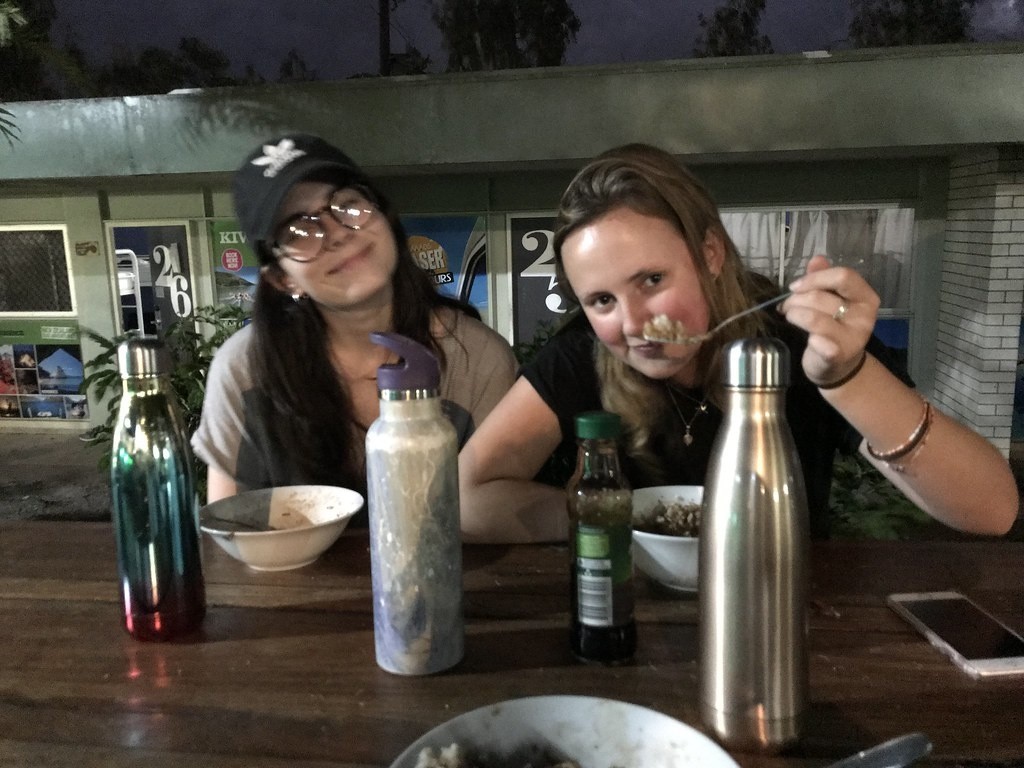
[628,498,701,538]
[415,743,584,768]
[642,314,688,342]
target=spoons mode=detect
[644,290,794,348]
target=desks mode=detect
[1,518,1024,768]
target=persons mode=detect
[208,133,524,523]
[457,142,1021,545]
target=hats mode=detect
[231,133,356,253]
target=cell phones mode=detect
[885,591,1024,682]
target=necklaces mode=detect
[665,382,714,448]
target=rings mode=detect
[833,299,853,323]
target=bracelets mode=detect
[819,351,868,390]
[867,396,935,473]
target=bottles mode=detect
[109,339,206,641]
[566,410,639,663]
[364,333,465,675]
[697,336,810,751]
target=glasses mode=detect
[268,184,375,264]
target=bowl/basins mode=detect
[202,484,364,571]
[630,485,703,591]
[388,694,741,768]
